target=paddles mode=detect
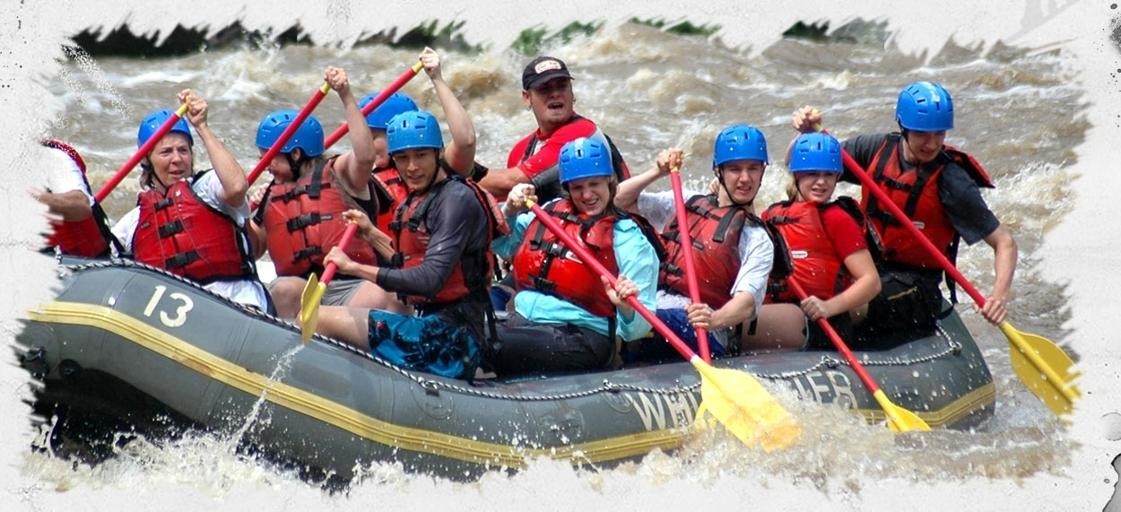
[667,166,722,431]
[524,194,800,453]
[811,114,1090,417]
[786,269,933,430]
[300,219,357,342]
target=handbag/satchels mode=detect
[367,305,482,384]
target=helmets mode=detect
[253,109,324,158]
[896,79,954,133]
[785,130,845,174]
[138,110,193,146]
[356,91,418,130]
[384,111,443,152]
[558,135,613,183]
[712,123,768,169]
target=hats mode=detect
[522,55,575,91]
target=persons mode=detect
[26,138,127,258]
[247,66,417,321]
[104,89,277,319]
[784,80,1018,326]
[484,137,660,380]
[471,56,638,215]
[708,129,883,350]
[614,124,776,362]
[251,47,477,268]
